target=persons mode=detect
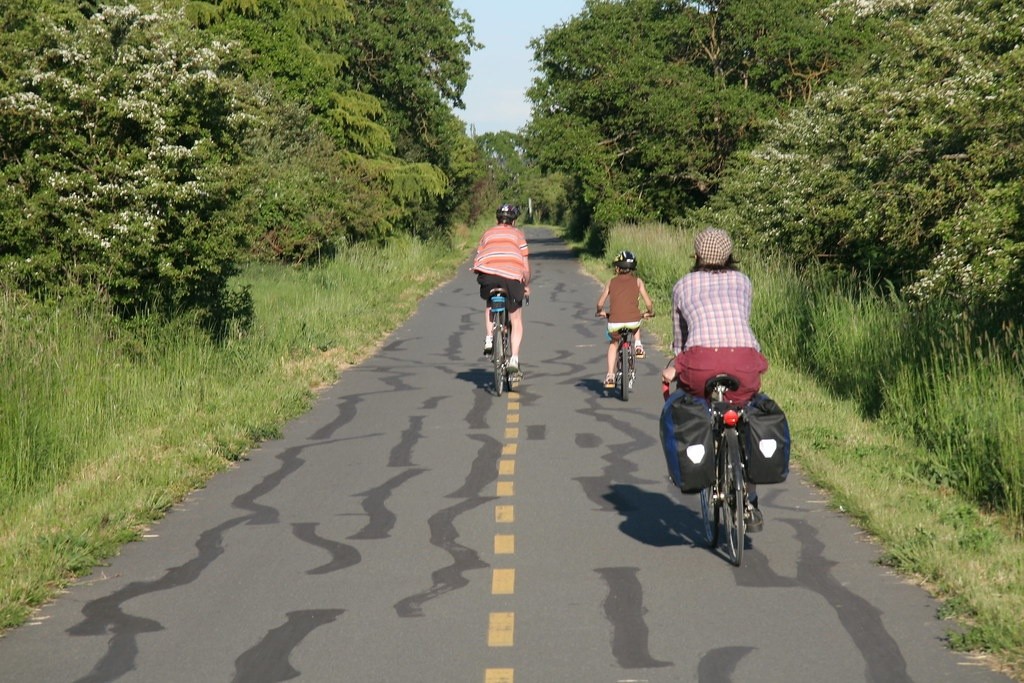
[663,228,768,532]
[597,251,653,388]
[473,204,530,378]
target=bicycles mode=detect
[483,287,529,397]
[659,357,791,567]
[595,312,655,401]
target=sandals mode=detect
[602,376,616,388]
[635,345,645,359]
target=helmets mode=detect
[612,251,637,269]
[496,204,520,220]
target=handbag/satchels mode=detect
[660,388,790,495]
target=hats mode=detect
[694,229,734,266]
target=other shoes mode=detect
[747,506,762,523]
[483,342,492,356]
[506,361,523,379]
